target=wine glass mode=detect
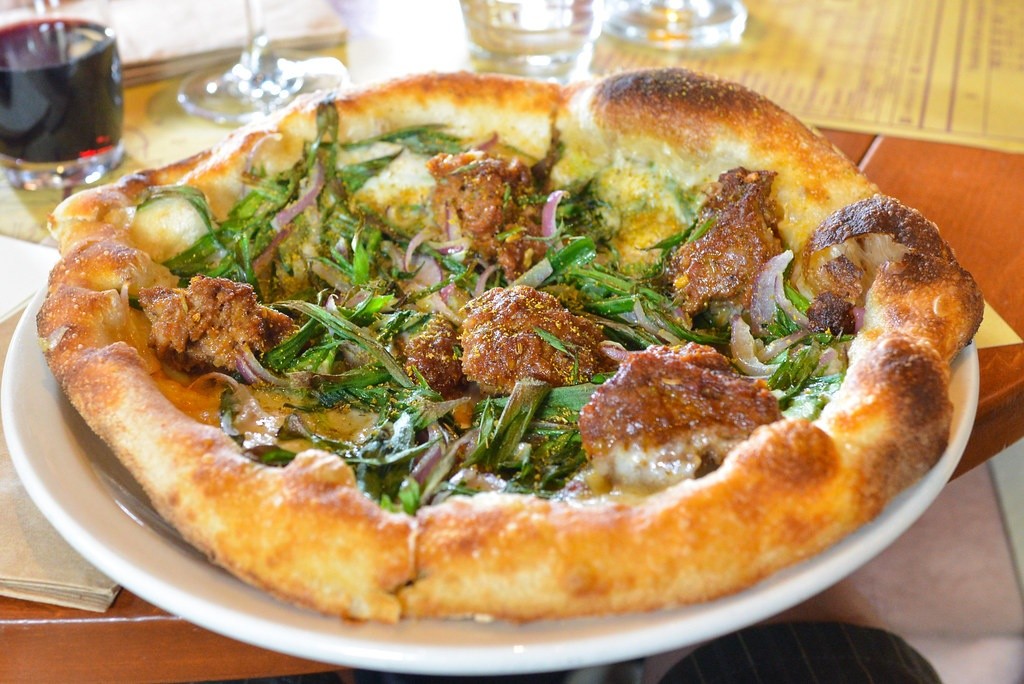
[176,0,352,127]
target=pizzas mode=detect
[35,66,983,621]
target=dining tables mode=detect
[0,0,1024,683]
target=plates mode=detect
[0,267,979,676]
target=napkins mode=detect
[0,234,128,615]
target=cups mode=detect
[0,1,126,191]
[602,2,747,54]
[459,0,602,83]
